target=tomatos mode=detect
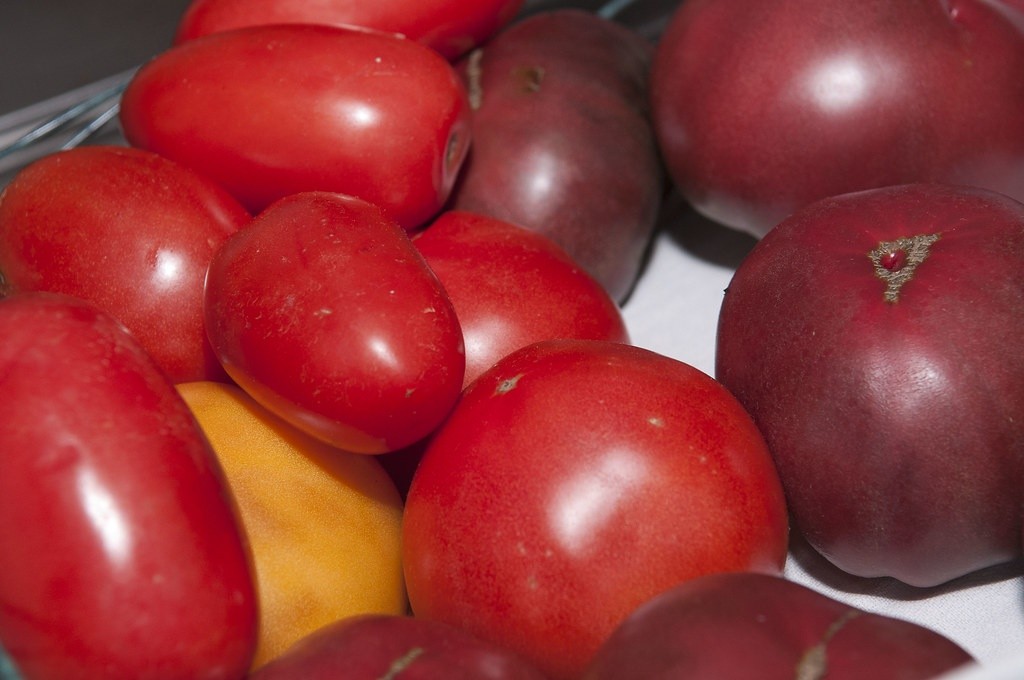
[0,1,1024,680]
